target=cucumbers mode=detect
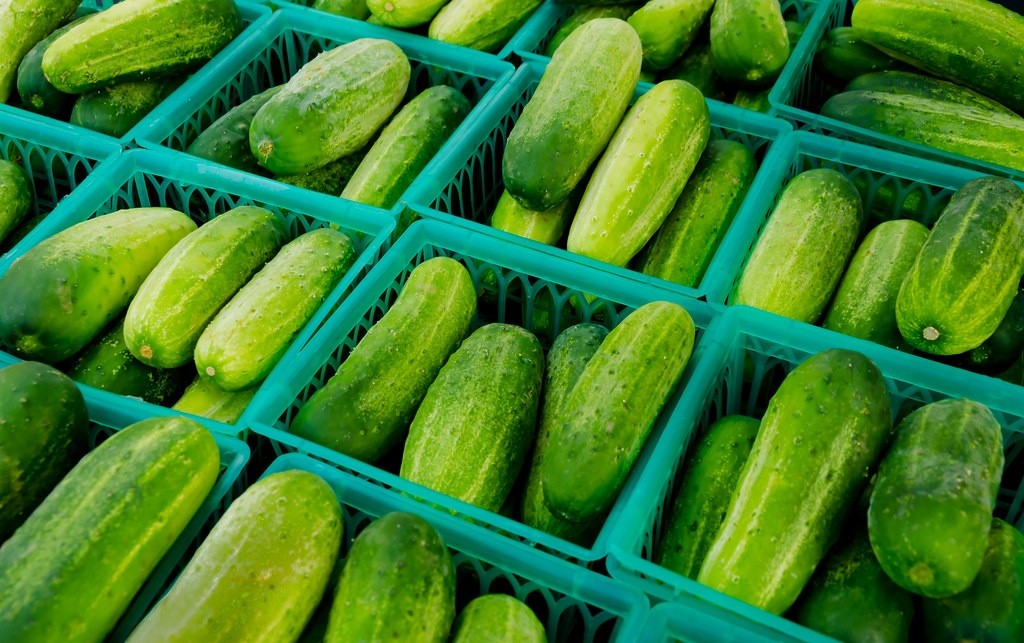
[1,0,1023,643]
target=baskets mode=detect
[0,0,1024,643]
